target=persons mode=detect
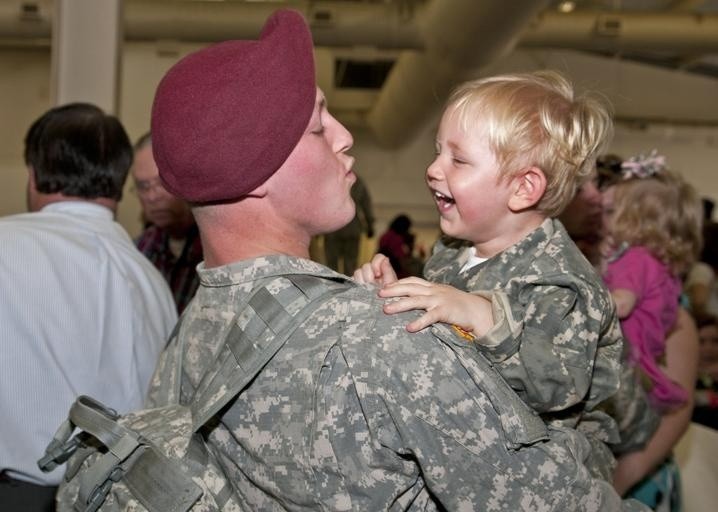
[130,130,204,317]
[348,71,632,479]
[381,213,427,279]
[2,101,182,510]
[139,11,653,512]
[308,172,377,278]
[554,153,718,510]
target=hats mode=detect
[150,9,316,206]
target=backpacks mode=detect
[38,274,352,512]
